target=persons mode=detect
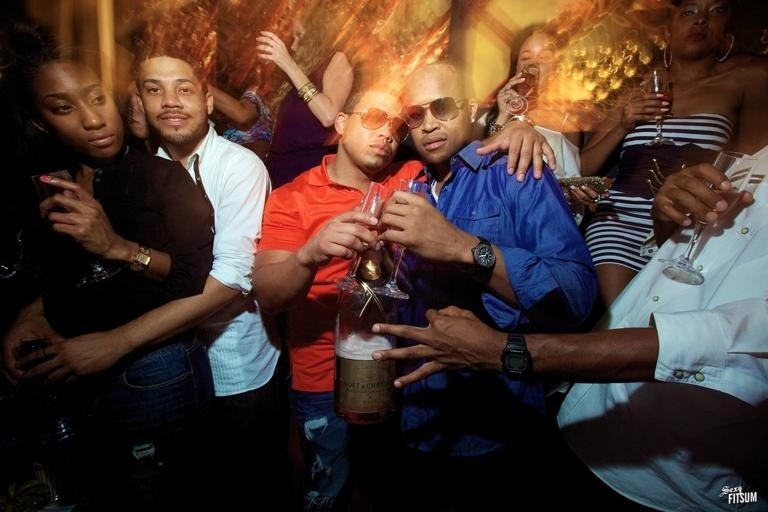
[2,0,768,510]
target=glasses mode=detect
[346,108,409,143]
[403,96,467,128]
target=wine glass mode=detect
[31,171,121,287]
[372,178,430,301]
[658,151,757,284]
[643,67,674,149]
[328,180,392,296]
[13,339,87,445]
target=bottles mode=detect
[332,254,400,424]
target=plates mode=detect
[557,176,617,195]
